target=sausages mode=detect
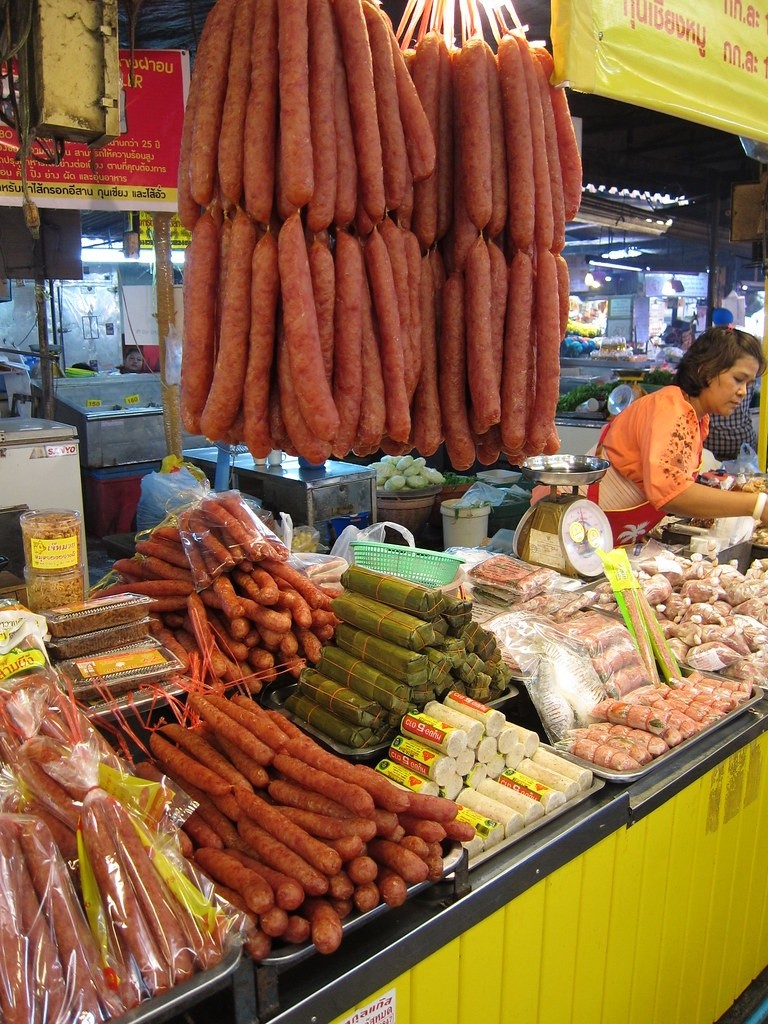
[176,0,583,470]
[92,492,344,698]
[0,639,477,1024]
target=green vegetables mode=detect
[556,381,617,414]
[641,368,677,389]
[442,472,489,484]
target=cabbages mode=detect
[367,453,445,491]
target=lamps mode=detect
[585,225,645,271]
[665,272,684,292]
[577,193,676,236]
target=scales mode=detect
[512,454,613,583]
[603,368,649,421]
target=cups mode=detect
[268,450,286,466]
[575,398,600,413]
[253,456,266,465]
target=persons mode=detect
[114,349,145,374]
[529,327,767,552]
[702,378,757,461]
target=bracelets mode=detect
[752,491,767,519]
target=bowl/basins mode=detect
[38,592,191,724]
[476,470,522,484]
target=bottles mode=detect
[19,508,83,571]
[23,564,85,611]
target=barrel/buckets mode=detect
[440,499,491,549]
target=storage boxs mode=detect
[318,512,369,547]
[83,462,160,534]
[477,469,523,484]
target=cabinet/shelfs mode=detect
[183,446,377,554]
